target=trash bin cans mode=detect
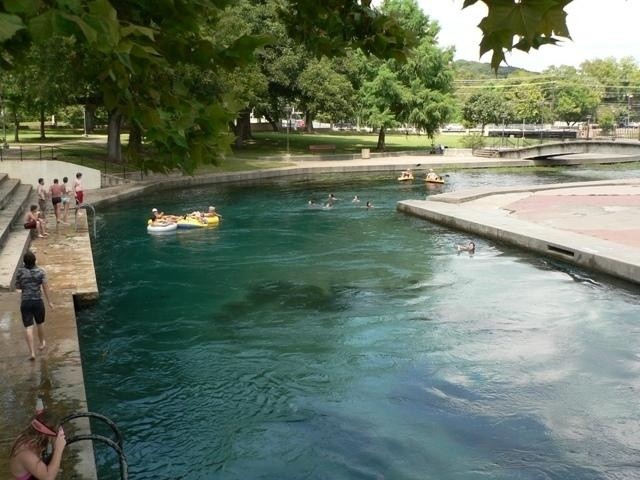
[361,148,370,159]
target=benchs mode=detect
[308,144,336,155]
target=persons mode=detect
[426,168,438,181]
[400,168,412,179]
[72,172,85,206]
[457,241,476,254]
[12,250,54,361]
[150,202,217,228]
[38,177,49,214]
[308,192,374,210]
[24,203,49,240]
[61,176,73,223]
[7,409,67,480]
[49,177,64,226]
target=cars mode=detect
[619,116,640,129]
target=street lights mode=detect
[586,114,592,141]
[500,111,507,145]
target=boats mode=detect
[146,212,220,233]
[399,172,414,181]
[425,174,445,183]
